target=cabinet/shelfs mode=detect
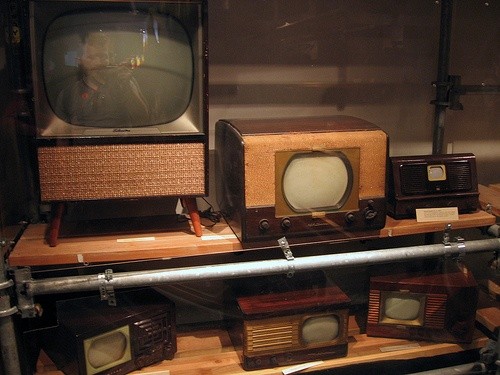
[1,206,500,375]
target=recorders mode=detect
[213,116,389,241]
[386,151,479,216]
[367,265,476,344]
[40,290,177,375]
[225,277,354,370]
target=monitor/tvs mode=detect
[29,1,208,140]
